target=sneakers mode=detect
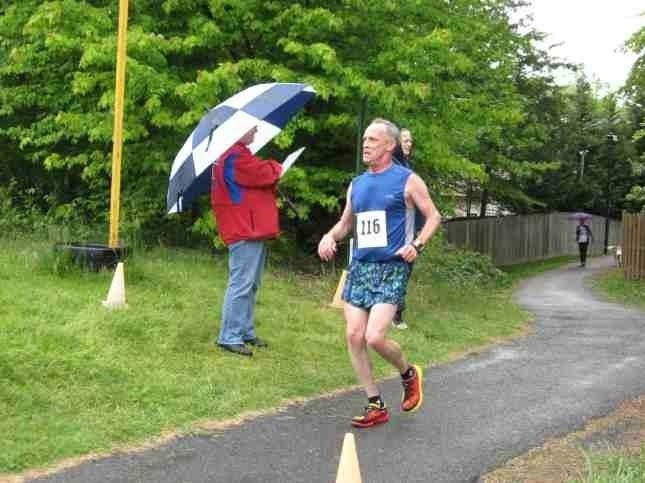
[351,400,389,427]
[400,362,423,411]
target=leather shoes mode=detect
[218,343,253,355]
[246,337,268,347]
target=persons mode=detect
[210,124,284,357]
[318,118,443,426]
[575,218,593,266]
[394,126,414,328]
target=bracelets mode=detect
[413,240,425,253]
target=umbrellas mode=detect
[167,82,317,222]
